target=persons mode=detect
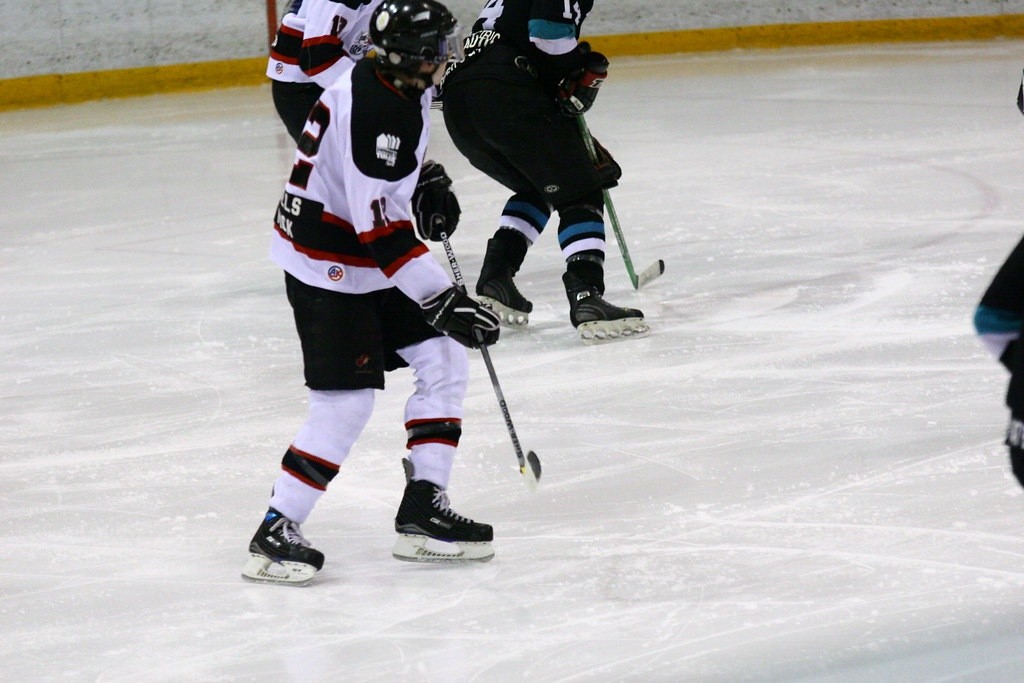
[243,0,497,584]
[975,71,1024,489]
[432,0,650,341]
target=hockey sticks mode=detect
[575,114,666,291]
[434,217,542,485]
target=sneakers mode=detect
[475,239,533,330]
[562,272,651,345]
[392,458,495,562]
[242,488,325,587]
[429,84,443,110]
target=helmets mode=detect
[370,0,465,76]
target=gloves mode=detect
[553,52,609,117]
[422,281,500,349]
[591,136,622,190]
[411,160,461,242]
